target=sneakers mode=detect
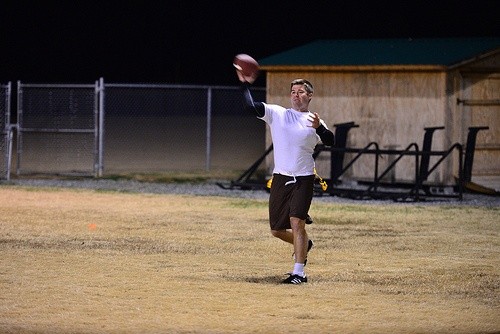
[303,239,313,267]
[280,274,307,284]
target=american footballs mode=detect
[233,54,259,76]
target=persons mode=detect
[237,69,335,284]
